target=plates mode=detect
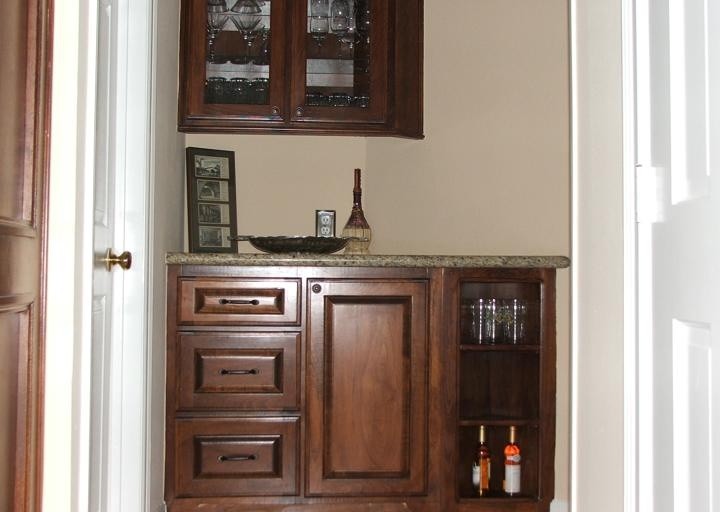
[246,234,356,254]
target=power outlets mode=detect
[315,209,336,238]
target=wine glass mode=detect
[310,17,329,48]
[331,17,350,48]
[207,14,229,62]
[230,14,271,62]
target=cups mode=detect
[311,0,329,17]
[470,297,527,345]
[231,0,265,14]
[331,0,349,17]
[207,0,228,14]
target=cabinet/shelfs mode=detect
[446,275,543,505]
[175,275,303,495]
[180,0,424,140]
[307,277,430,499]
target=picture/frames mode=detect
[184,146,238,252]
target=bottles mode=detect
[500,425,523,497]
[472,424,492,497]
